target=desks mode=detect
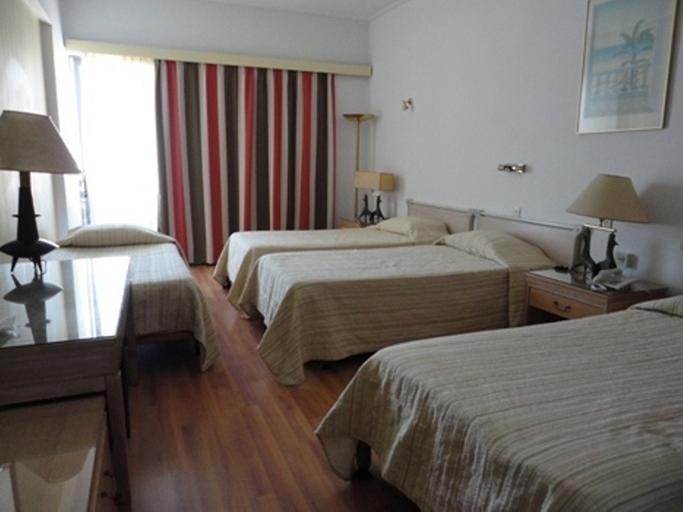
[0,254,132,512]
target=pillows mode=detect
[627,294,683,317]
[57,225,188,267]
[432,228,559,328]
[374,215,450,243]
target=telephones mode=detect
[592,268,641,290]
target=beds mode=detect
[212,198,477,320]
[316,294,683,511]
[0,223,219,379]
[238,214,584,386]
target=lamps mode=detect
[496,163,528,172]
[0,109,80,272]
[343,113,374,225]
[353,170,395,226]
[3,265,63,349]
[565,174,651,296]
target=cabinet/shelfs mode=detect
[0,394,123,512]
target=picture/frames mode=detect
[575,1,680,134]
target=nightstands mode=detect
[334,215,368,228]
[520,266,668,327]
